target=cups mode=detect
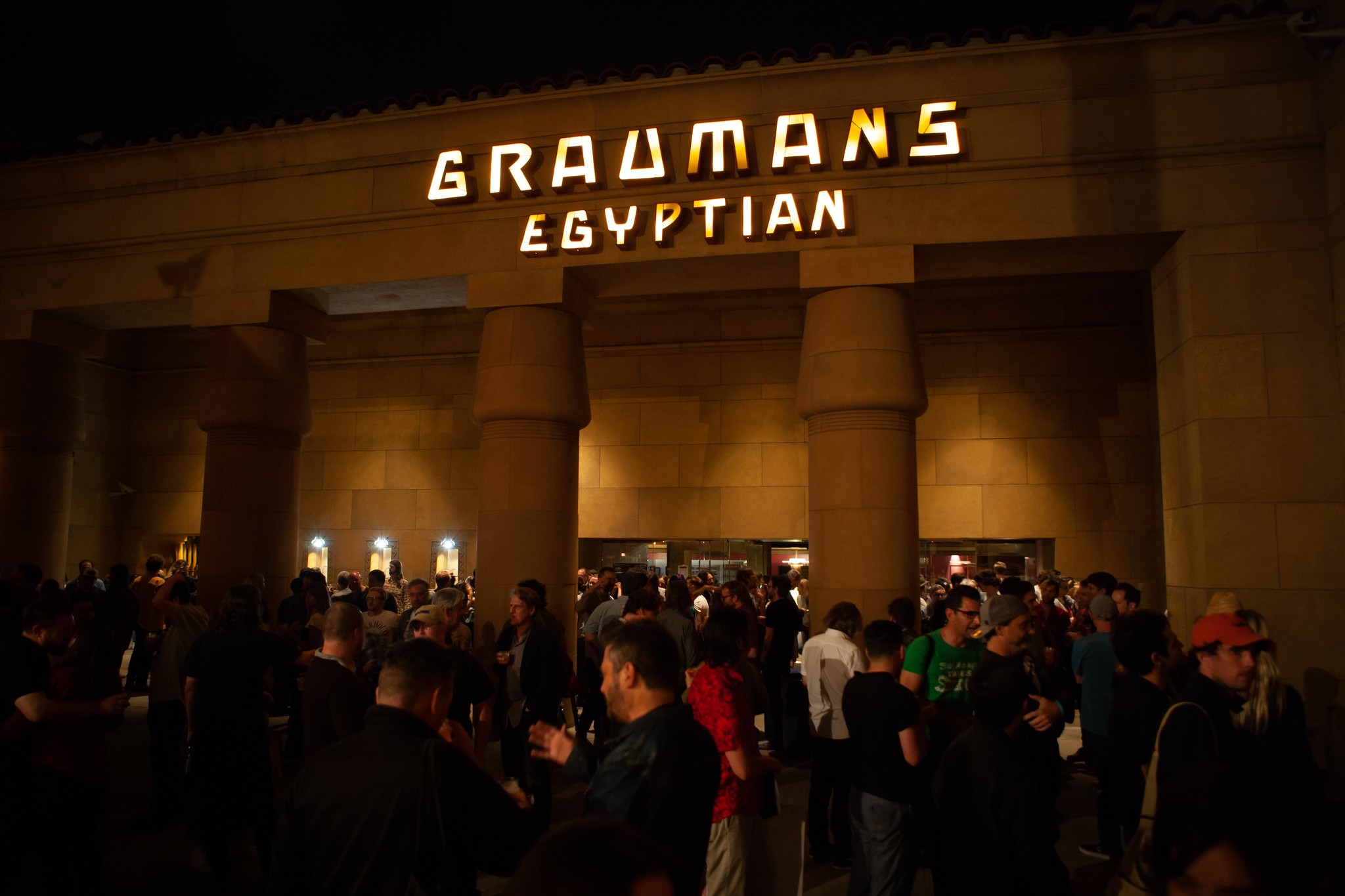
[499,651,510,662]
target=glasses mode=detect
[366,596,383,601]
[722,594,736,601]
[950,608,980,619]
[767,585,774,589]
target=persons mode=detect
[899,584,984,737]
[358,586,403,666]
[493,585,561,831]
[799,601,868,869]
[0,559,1345,896]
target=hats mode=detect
[1089,594,1117,621]
[83,568,99,578]
[669,574,686,583]
[409,604,445,629]
[1192,613,1266,647]
[919,574,929,586]
[970,594,1029,638]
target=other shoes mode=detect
[124,684,150,697]
[758,741,770,750]
[757,730,766,741]
[128,642,135,649]
